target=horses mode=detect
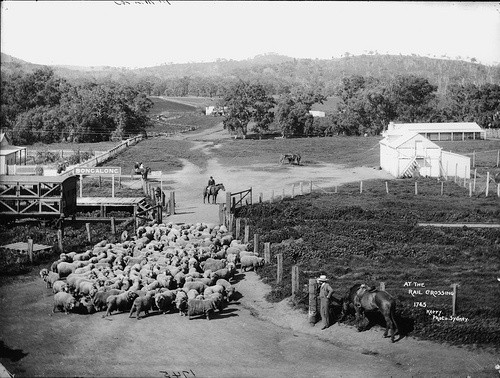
[202,183,226,205]
[279,153,301,166]
[130,166,152,181]
[338,283,401,344]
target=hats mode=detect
[318,275,329,281]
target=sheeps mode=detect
[39,222,265,320]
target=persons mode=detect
[134,161,139,169]
[139,162,144,169]
[204,175,217,188]
[315,272,333,331]
